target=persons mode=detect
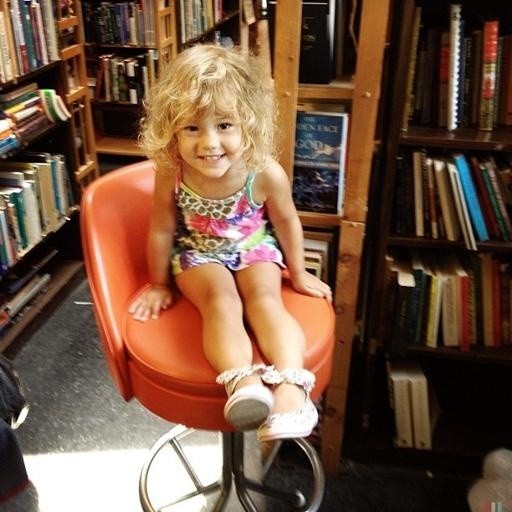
[127,44,333,442]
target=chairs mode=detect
[76,158,336,512]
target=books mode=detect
[390,367,432,448]
[414,150,512,250]
[0,0,75,334]
[292,110,349,214]
[81,0,222,105]
[302,230,334,281]
[401,5,512,133]
[267,0,345,85]
[377,249,512,350]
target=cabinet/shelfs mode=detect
[2,0,100,366]
[82,0,272,157]
[274,1,512,472]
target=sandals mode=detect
[215,364,319,443]
